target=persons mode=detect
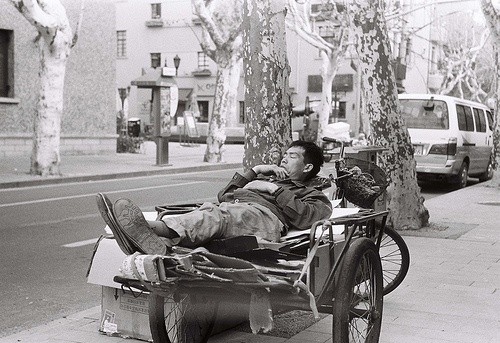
[95,140,334,258]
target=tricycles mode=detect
[110,137,412,343]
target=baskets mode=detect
[336,156,391,208]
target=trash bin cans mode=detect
[327,147,390,225]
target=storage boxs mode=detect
[84,231,219,342]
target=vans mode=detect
[396,92,498,192]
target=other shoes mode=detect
[96,192,138,255]
[113,198,167,258]
